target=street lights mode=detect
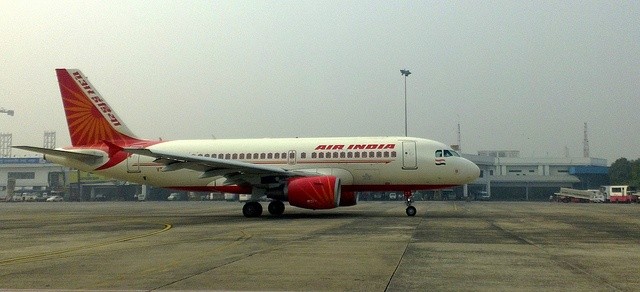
[400,69,412,137]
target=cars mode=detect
[168,193,181,201]
[47,195,64,202]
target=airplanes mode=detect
[8,68,482,222]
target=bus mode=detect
[610,184,637,204]
